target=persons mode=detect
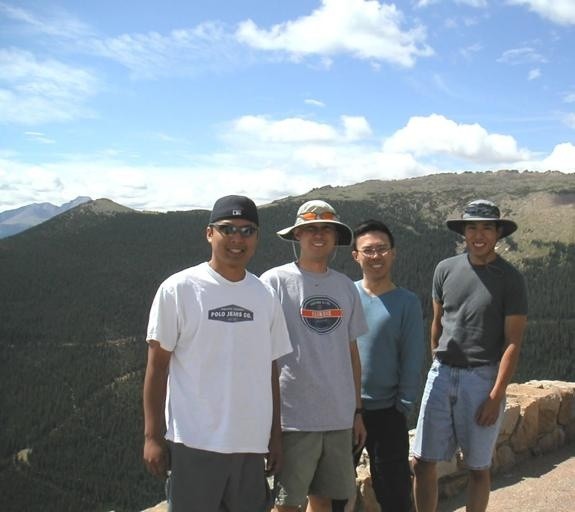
[334,219,422,512]
[411,198,530,512]
[140,195,292,510]
[258,198,371,512]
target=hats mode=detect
[276,200,353,246]
[210,196,258,225]
[446,201,517,238]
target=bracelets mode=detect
[355,407,366,415]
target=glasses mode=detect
[209,223,256,236]
[357,247,391,254]
[296,212,335,220]
